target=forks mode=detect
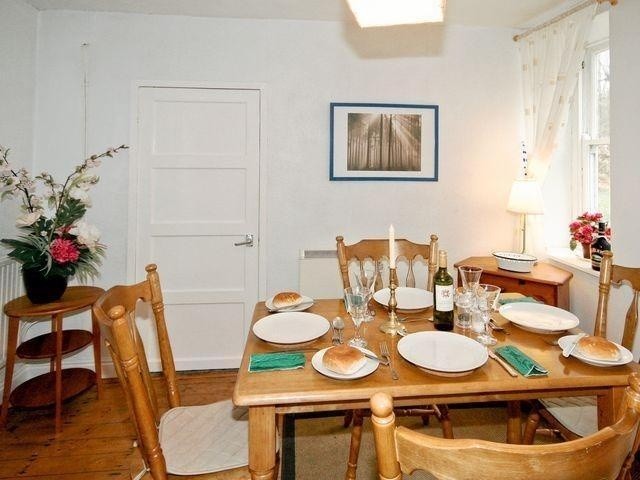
[330,317,340,346]
[380,340,394,379]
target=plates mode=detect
[252,313,330,346]
[263,294,315,312]
[557,334,635,367]
[396,331,489,378]
[311,345,379,379]
[499,302,580,334]
[372,286,436,313]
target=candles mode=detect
[389,224,395,268]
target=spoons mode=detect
[334,314,344,345]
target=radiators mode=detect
[0,255,24,370]
[299,247,384,299]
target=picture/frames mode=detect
[329,102,439,182]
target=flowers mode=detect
[0,142,130,287]
[568,211,611,251]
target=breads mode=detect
[272,292,302,307]
[322,344,367,374]
[579,336,622,362]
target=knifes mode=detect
[486,349,518,378]
[563,333,586,357]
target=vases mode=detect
[22,262,68,304]
[581,242,592,259]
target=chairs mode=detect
[93,263,281,480]
[522,250,640,445]
[336,234,455,480]
[371,372,640,480]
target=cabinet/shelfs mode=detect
[453,257,573,312]
[0,286,105,434]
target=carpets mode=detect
[277,400,566,480]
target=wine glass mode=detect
[454,266,501,345]
[344,269,378,347]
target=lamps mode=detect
[344,0,447,29]
[506,176,544,266]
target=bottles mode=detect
[433,250,454,331]
[589,222,612,271]
